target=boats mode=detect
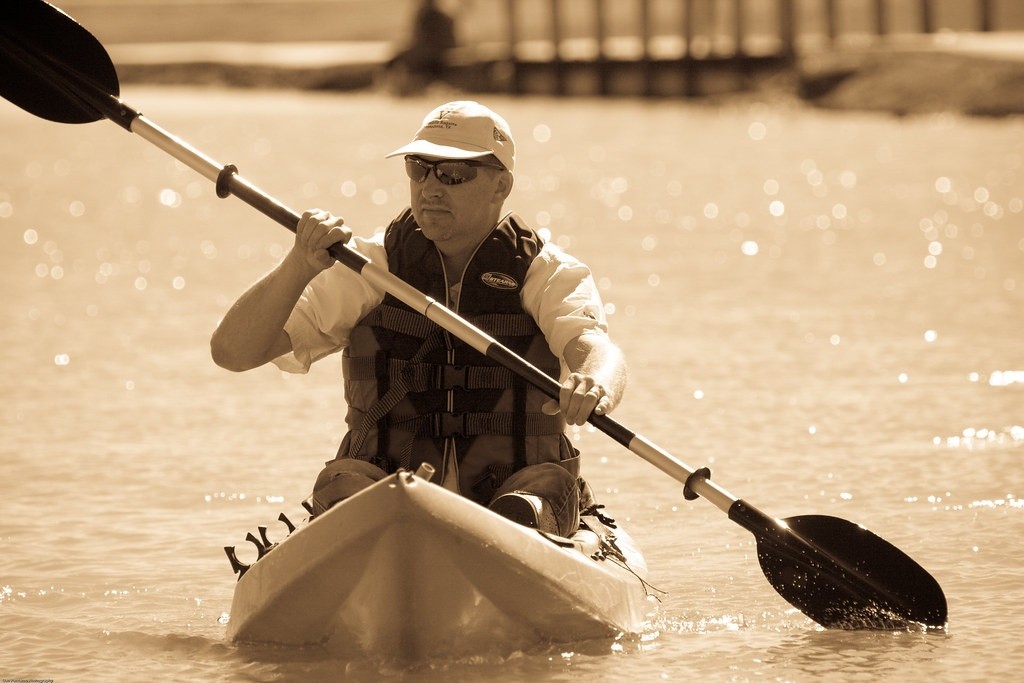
[221,466,651,650]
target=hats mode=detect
[385,101,515,175]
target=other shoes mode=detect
[491,492,540,530]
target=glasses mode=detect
[404,155,504,186]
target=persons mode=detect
[210,101,629,541]
[383,1,454,81]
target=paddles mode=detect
[0,0,950,633]
